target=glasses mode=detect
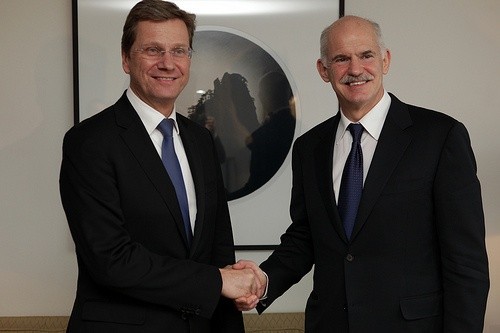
[126,44,192,62]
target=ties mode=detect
[339,123,364,241]
[156,119,190,247]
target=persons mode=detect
[60,1,262,333]
[233,15,490,333]
